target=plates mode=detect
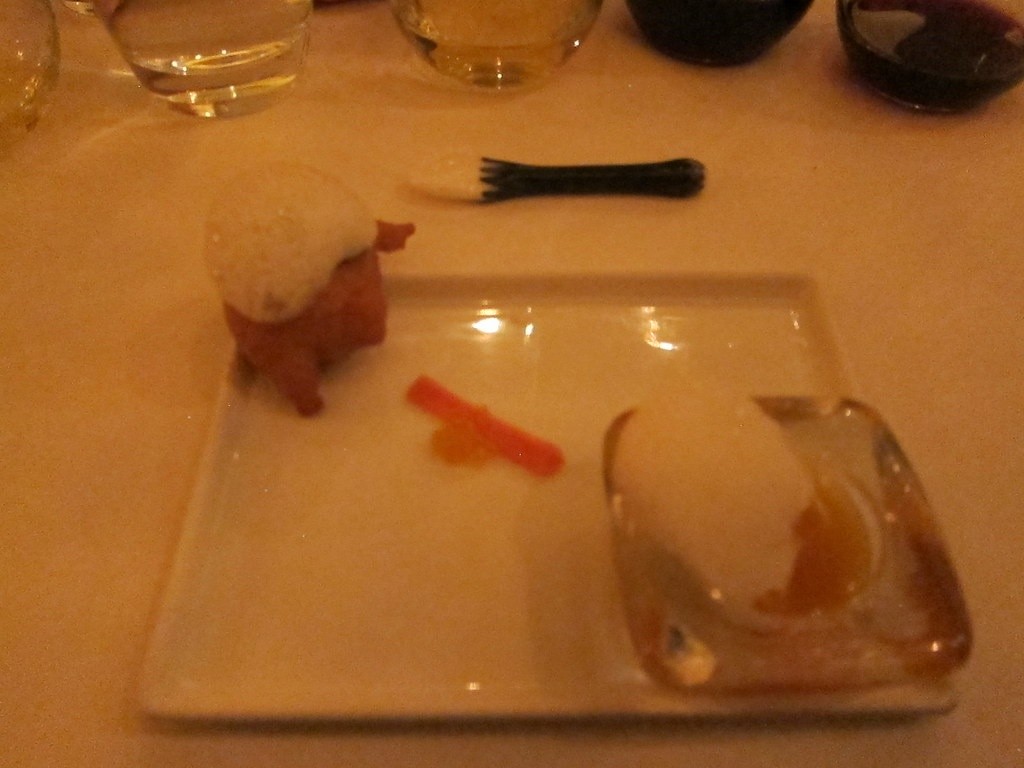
[136,266,958,721]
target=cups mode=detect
[835,0,1024,113]
[622,0,816,68]
[92,0,313,119]
[390,0,603,89]
[0,0,60,146]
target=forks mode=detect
[478,155,706,201]
